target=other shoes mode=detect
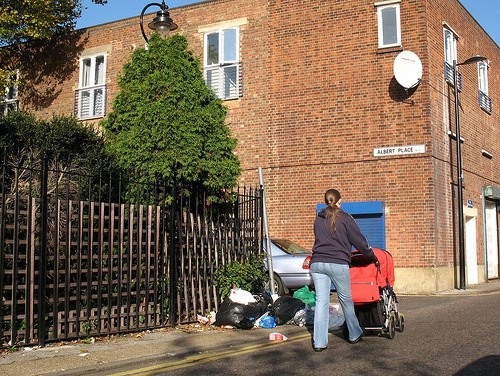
[349,335,362,344]
[315,347,327,352]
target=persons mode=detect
[307,189,378,351]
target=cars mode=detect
[262,236,313,297]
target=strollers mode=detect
[349,247,405,341]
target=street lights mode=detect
[452,55,487,287]
